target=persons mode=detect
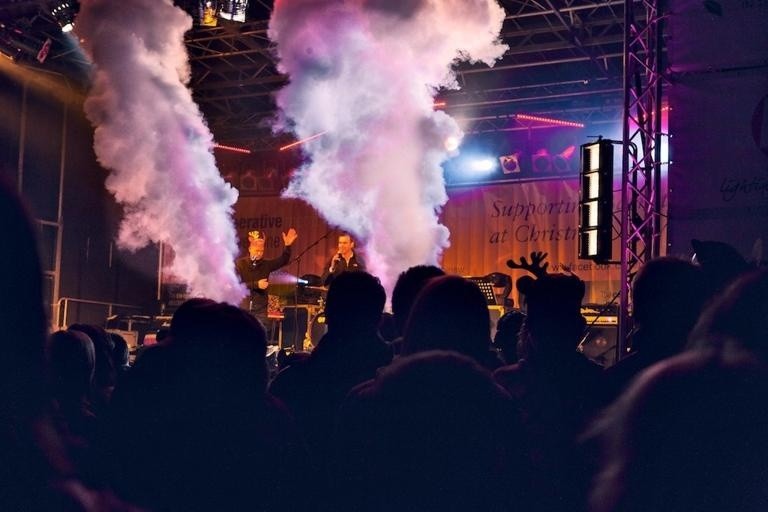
[0,239,768,512]
[321,231,366,288]
[3,180,124,512]
[235,227,298,319]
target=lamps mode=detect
[198,0,217,27]
[495,143,576,174]
[216,0,248,24]
[49,0,76,33]
[30,12,58,41]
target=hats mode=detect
[516,274,584,303]
[155,297,266,355]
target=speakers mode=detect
[580,325,617,369]
[281,305,308,350]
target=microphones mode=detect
[335,250,342,264]
